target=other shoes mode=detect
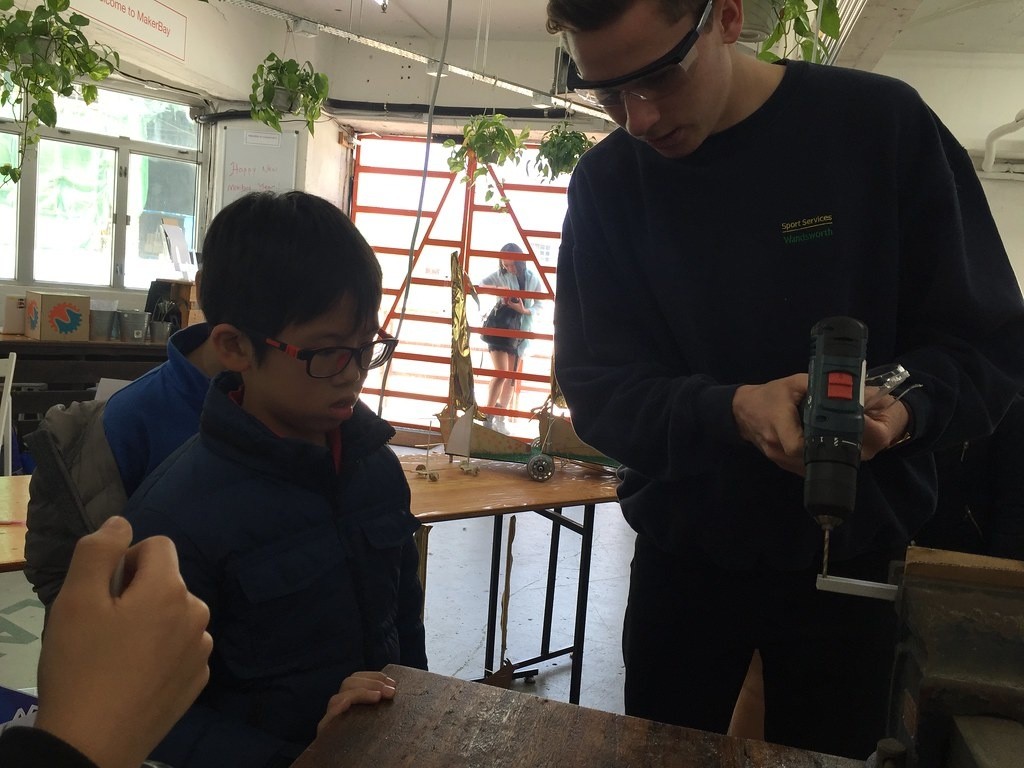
[482,418,493,432]
[494,418,510,435]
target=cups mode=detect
[148,320,172,344]
[117,312,152,343]
[90,310,116,341]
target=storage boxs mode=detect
[25,291,91,342]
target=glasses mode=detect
[237,323,401,379]
[565,0,713,107]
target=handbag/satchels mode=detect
[482,304,522,350]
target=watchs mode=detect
[886,398,914,452]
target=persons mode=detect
[19,192,429,767]
[1,513,213,768]
[546,0,1024,761]
[475,243,544,434]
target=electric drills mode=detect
[789,308,871,580]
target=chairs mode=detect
[0,353,16,476]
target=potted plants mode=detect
[442,112,530,214]
[736,0,840,61]
[533,122,597,186]
[0,0,119,189]
[250,52,329,139]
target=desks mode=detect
[0,440,624,704]
[291,663,890,768]
[0,332,168,362]
